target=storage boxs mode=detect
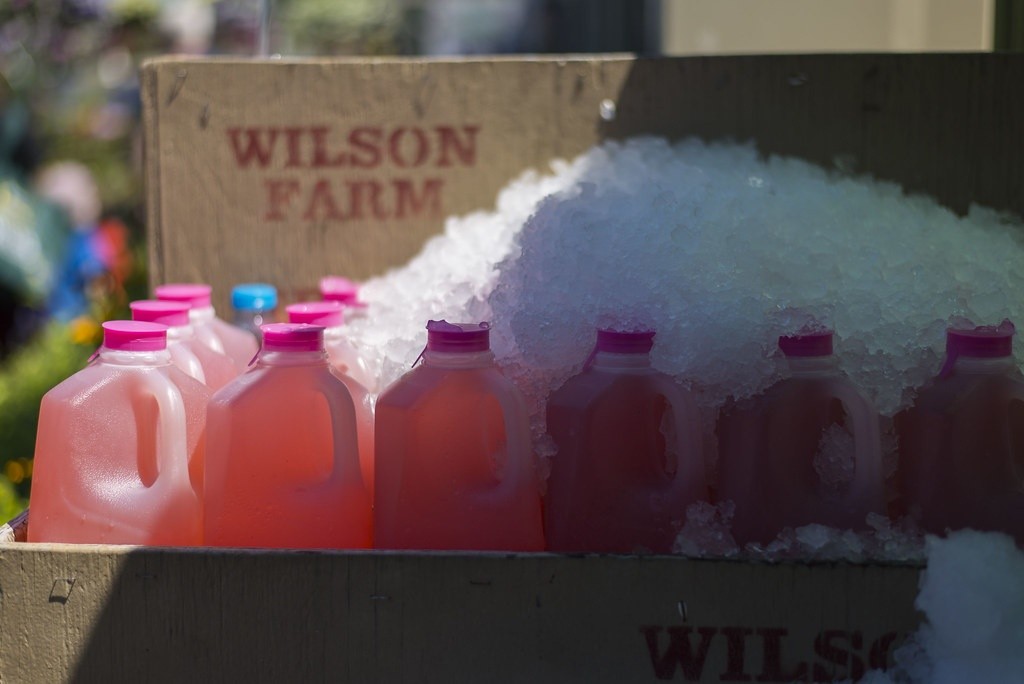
[0,47,1024,682]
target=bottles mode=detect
[714,328,884,552]
[892,327,1024,548]
[372,320,544,552]
[543,327,708,555]
[23,275,371,549]
